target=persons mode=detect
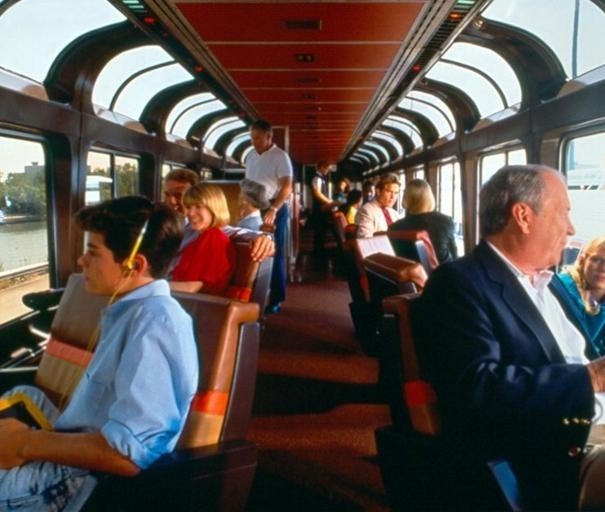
[362,181,376,204]
[557,236,604,358]
[388,180,457,265]
[415,164,604,511]
[339,189,363,224]
[168,182,237,295]
[245,121,294,313]
[163,169,276,281]
[354,172,399,239]
[0,195,198,511]
[332,179,350,203]
[237,179,270,231]
[311,160,338,262]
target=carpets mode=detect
[381,205,394,226]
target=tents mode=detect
[122,216,150,272]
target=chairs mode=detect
[265,303,282,314]
[324,193,604,510]
[2,192,308,512]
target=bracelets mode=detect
[270,206,277,211]
